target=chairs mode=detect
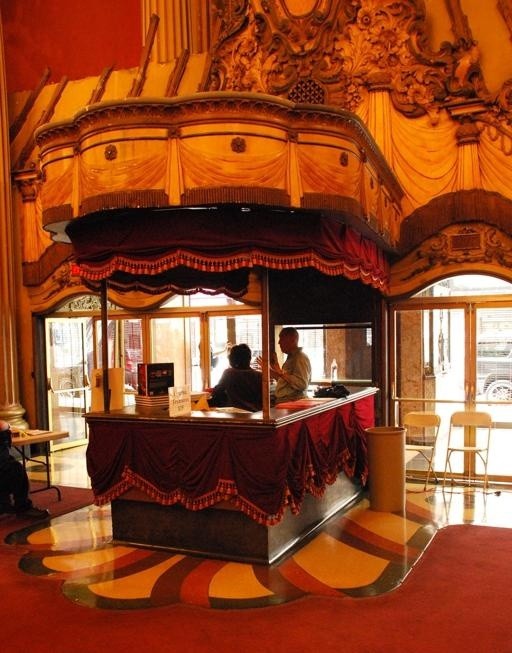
[445,411,494,492]
[402,412,442,491]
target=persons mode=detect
[205,341,262,412]
[255,326,312,405]
[1,420,50,521]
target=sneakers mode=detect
[14,507,49,520]
[0,503,17,514]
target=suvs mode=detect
[476,331,512,402]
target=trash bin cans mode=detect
[364,426,407,512]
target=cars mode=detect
[53,344,86,400]
[111,349,142,389]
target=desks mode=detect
[0,426,69,503]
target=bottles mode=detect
[331,359,338,380]
